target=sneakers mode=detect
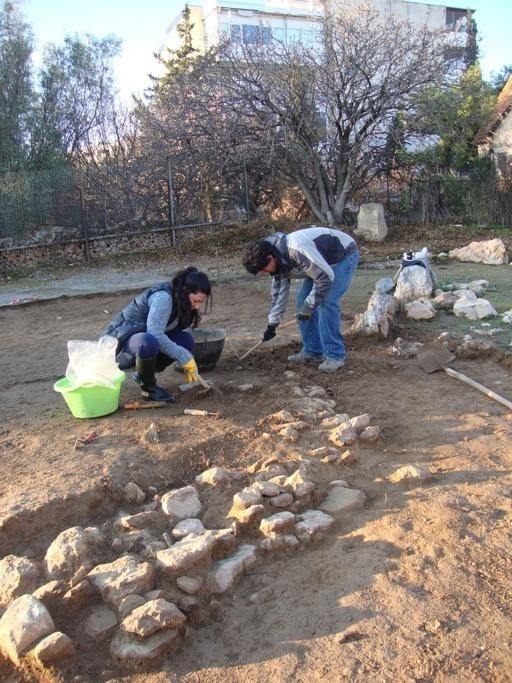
[318,354,346,375]
[133,371,176,403]
[286,351,319,368]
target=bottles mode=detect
[403,245,428,260]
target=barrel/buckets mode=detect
[358,204,388,242]
[174,327,224,371]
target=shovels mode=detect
[417,342,512,410]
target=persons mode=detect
[242,227,359,373]
[99,266,213,404]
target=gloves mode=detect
[296,303,314,320]
[261,324,279,342]
[182,358,203,386]
